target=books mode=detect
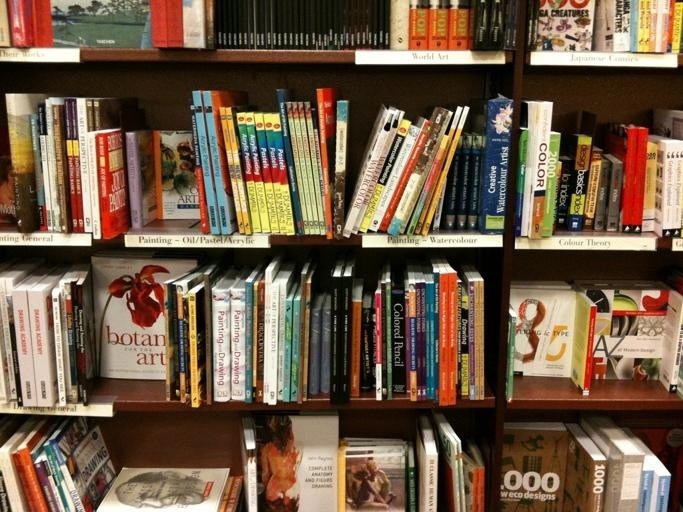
[506,279,680,403]
[500,415,680,512]
[255,411,467,512]
[2,416,258,512]
[343,93,514,238]
[2,253,484,405]
[2,93,130,240]
[2,2,212,49]
[526,3,681,54]
[125,87,349,240]
[514,100,680,239]
[462,442,485,512]
[215,3,516,51]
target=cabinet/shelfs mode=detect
[0,0,683,512]
